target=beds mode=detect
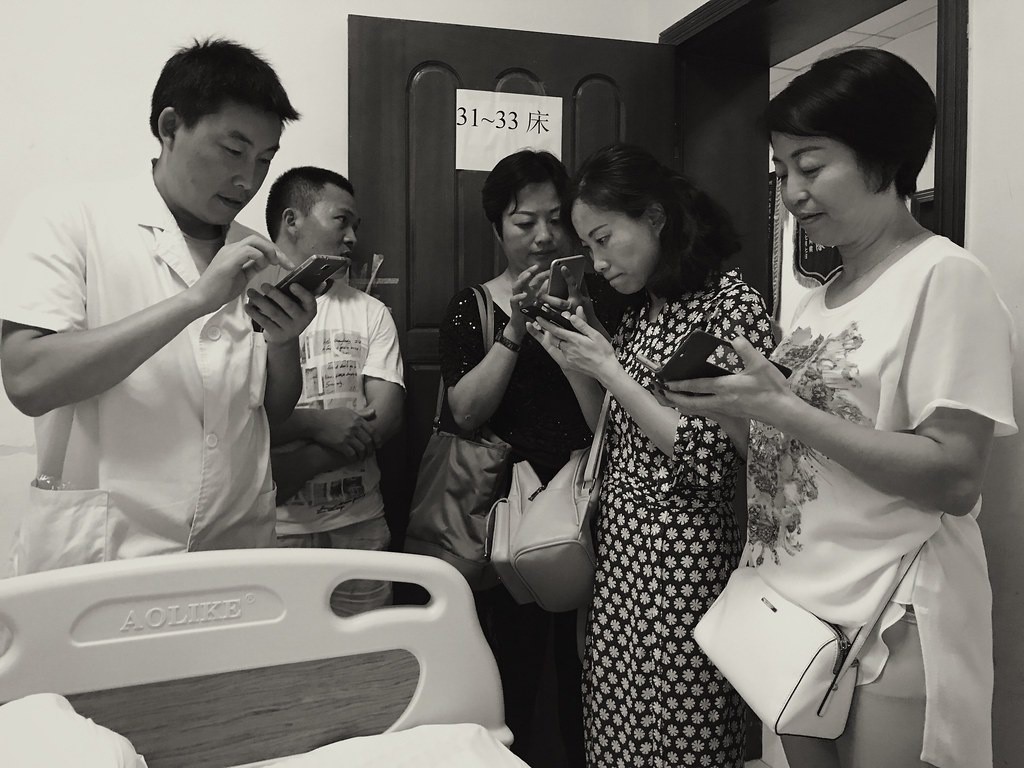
[0,547,528,768]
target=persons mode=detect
[526,149,777,768]
[643,48,1018,768]
[265,166,405,614]
[0,40,318,559]
[441,152,618,767]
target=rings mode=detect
[557,340,563,350]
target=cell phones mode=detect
[248,253,352,310]
[548,254,586,301]
[636,328,794,398]
[520,299,583,336]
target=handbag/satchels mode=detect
[404,285,517,594]
[694,565,861,741]
[485,389,613,614]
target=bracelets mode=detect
[495,328,523,353]
